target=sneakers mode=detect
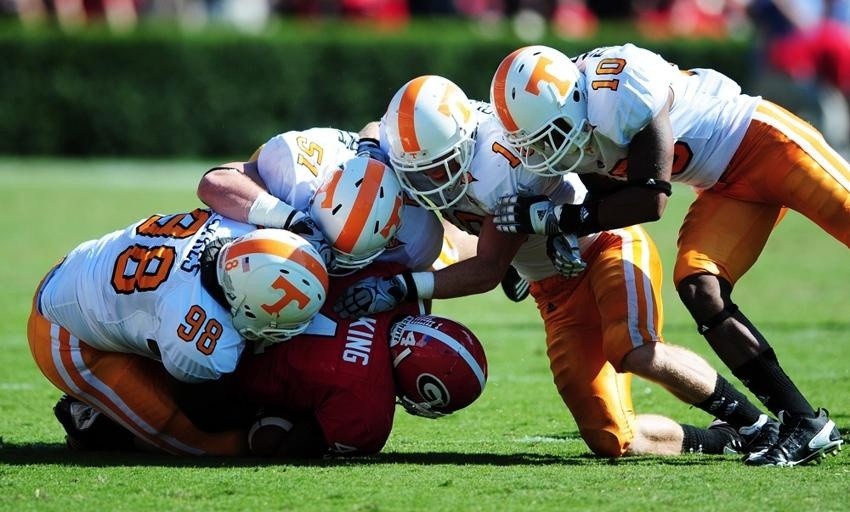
[54,393,104,445]
[707,415,740,455]
[743,409,842,467]
[740,414,781,458]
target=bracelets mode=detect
[396,271,435,300]
[579,198,598,231]
[249,187,295,230]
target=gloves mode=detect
[546,234,587,277]
[333,271,418,318]
[289,217,336,272]
[494,194,561,235]
[358,146,388,165]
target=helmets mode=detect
[312,157,404,277]
[216,229,329,343]
[390,315,486,418]
[387,74,476,210]
[490,45,591,177]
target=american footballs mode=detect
[248,408,293,460]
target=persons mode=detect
[333,70,778,458]
[27,201,329,462]
[491,32,850,463]
[198,125,460,273]
[50,273,488,458]
